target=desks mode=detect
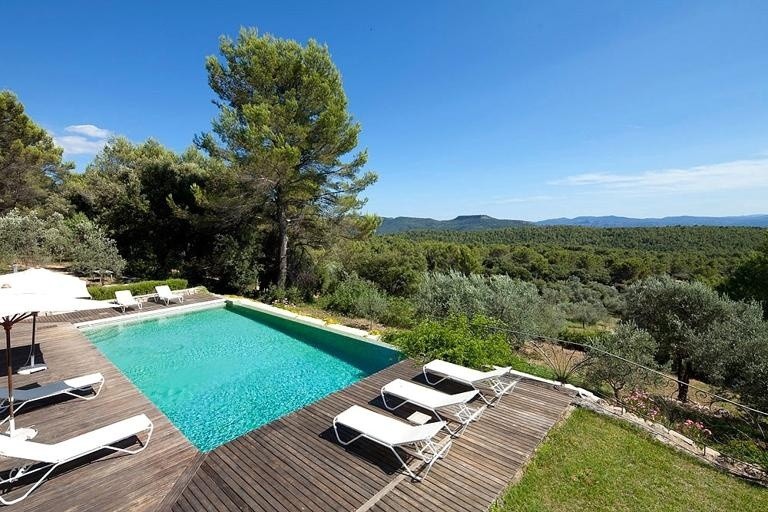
[92,270,113,284]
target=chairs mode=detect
[114,289,143,312]
[333,357,518,482]
[154,285,183,306]
[0,371,154,504]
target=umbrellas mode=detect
[0,268,92,357]
[0,282,123,420]
